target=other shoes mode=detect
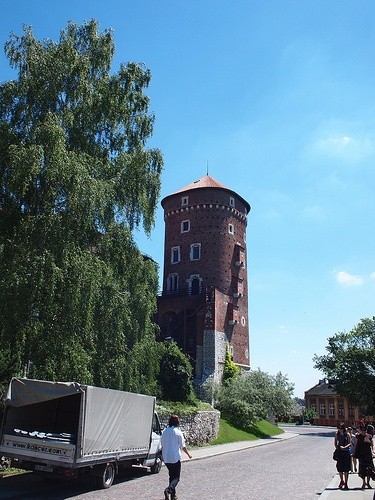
[343,483,348,490]
[361,482,366,489]
[350,471,354,474]
[164,488,170,500]
[171,495,179,500]
[354,468,357,472]
[339,481,344,489]
[365,483,372,489]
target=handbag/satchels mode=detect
[333,447,339,461]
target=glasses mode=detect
[337,426,344,429]
[361,428,366,431]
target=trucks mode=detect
[0,376,162,488]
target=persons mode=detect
[160,415,192,500]
[333,418,375,490]
